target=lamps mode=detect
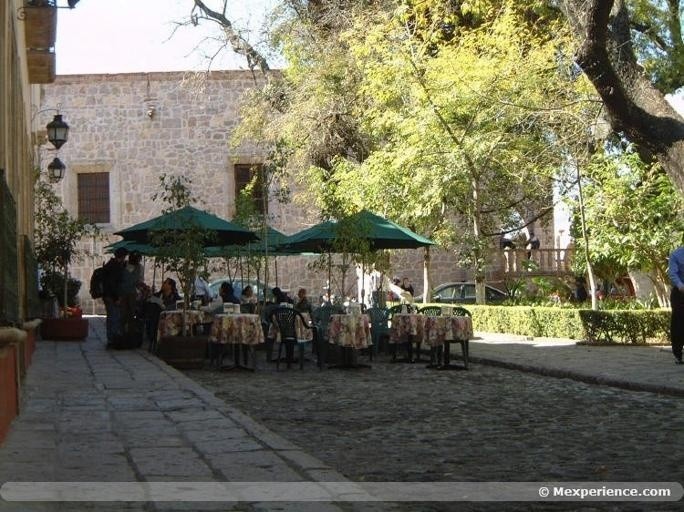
[31,106,70,184]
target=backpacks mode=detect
[117,263,141,297]
[90,260,114,299]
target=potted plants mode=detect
[151,222,206,372]
[38,217,101,341]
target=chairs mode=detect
[262,301,473,371]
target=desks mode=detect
[160,310,262,373]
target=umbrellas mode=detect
[301,208,435,311]
[269,219,338,303]
[102,238,206,285]
[221,226,301,302]
[113,207,261,299]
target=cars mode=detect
[414,281,519,307]
[208,277,286,306]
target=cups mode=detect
[233,304,240,313]
[401,305,408,314]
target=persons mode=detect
[575,281,587,303]
[554,292,562,302]
[595,286,628,300]
[669,233,684,364]
[89,249,414,349]
